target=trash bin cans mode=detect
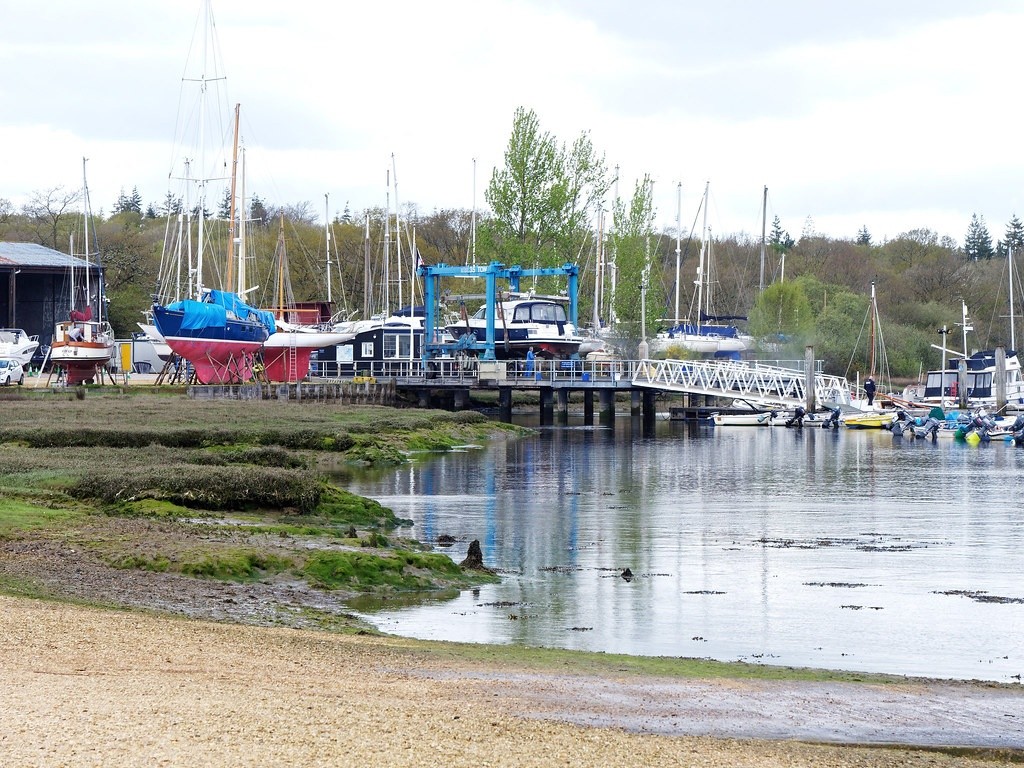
[582,373,590,382]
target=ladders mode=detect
[113,344,126,384]
[289,311,298,383]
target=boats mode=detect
[0,329,39,367]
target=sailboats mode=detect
[50,157,115,385]
[137,0,1024,447]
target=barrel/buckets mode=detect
[954,429,966,438]
[535,372,542,381]
[581,373,589,382]
[966,432,981,441]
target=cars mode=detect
[0,358,24,387]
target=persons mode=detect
[864,376,876,406]
[69,327,86,341]
[524,346,536,377]
